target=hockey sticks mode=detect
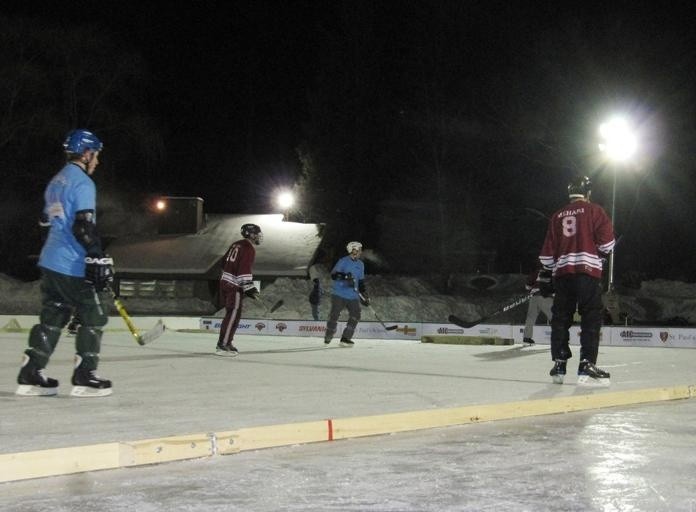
[448,291,536,330]
[354,288,399,331]
[257,295,285,316]
[105,286,167,346]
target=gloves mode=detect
[361,294,370,307]
[86,248,113,293]
[596,247,612,267]
[539,270,556,298]
[241,281,260,299]
[344,273,353,280]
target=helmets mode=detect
[241,224,263,245]
[64,129,103,153]
[347,242,362,253]
[568,175,592,197]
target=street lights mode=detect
[597,115,632,292]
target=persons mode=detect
[533,174,617,378]
[324,242,370,346]
[213,223,264,354]
[14,129,114,389]
[67,307,82,336]
[523,264,558,346]
[307,276,322,322]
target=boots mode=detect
[72,352,111,388]
[17,348,57,387]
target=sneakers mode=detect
[325,337,330,343]
[523,337,534,343]
[550,359,566,376]
[578,360,609,378]
[341,337,354,344]
[216,341,238,351]
